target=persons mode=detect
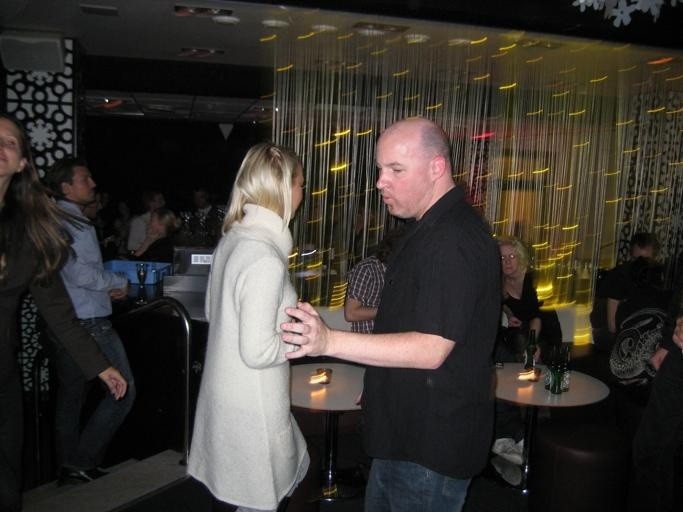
[278,116,504,512]
[134,208,182,260]
[83,190,104,220]
[491,237,543,465]
[182,186,229,248]
[631,249,683,512]
[1,116,129,511]
[47,156,130,487]
[104,201,128,257]
[183,142,315,511]
[603,234,659,333]
[605,257,667,406]
[128,190,167,251]
[342,235,398,334]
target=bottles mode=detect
[524,329,536,369]
[543,342,573,395]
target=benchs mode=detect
[314,303,575,369]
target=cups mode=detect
[133,287,148,305]
[316,368,332,384]
[135,263,149,288]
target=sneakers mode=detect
[488,456,524,489]
[489,438,523,467]
[56,468,108,483]
